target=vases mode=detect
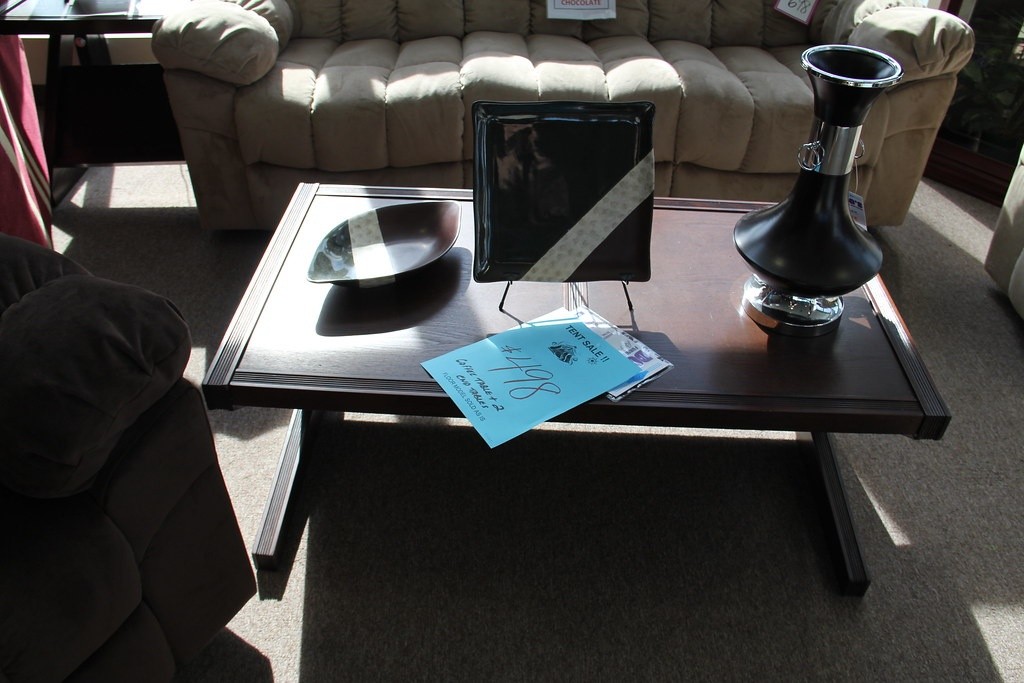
[731,44,904,338]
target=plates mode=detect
[470,99,656,283]
[305,199,461,290]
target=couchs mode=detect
[0,228,258,683]
[152,0,973,234]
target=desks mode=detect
[1,0,167,201]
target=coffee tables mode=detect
[200,183,951,594]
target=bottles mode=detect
[733,44,903,298]
